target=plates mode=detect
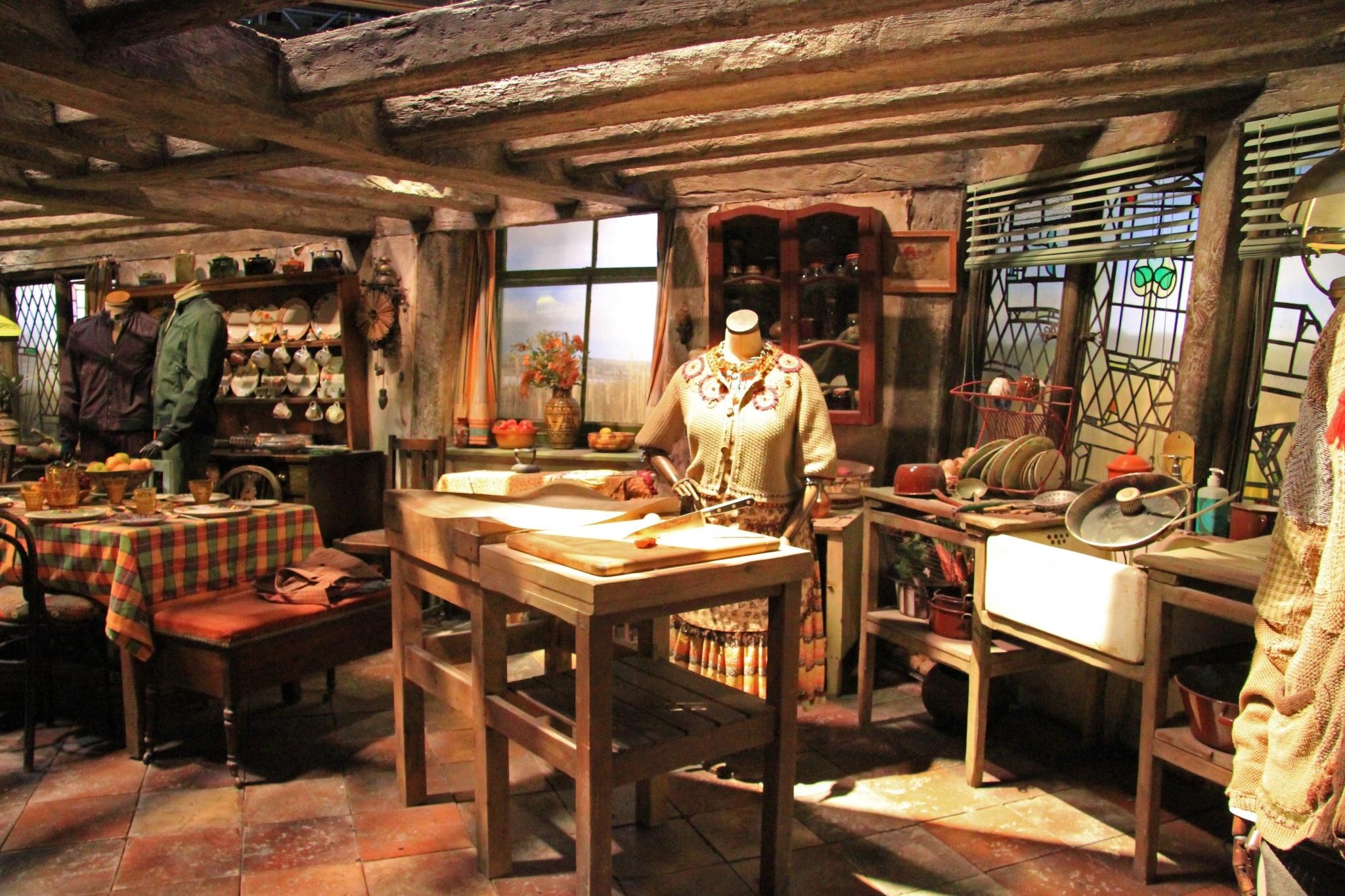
[0,493,280,526]
[954,434,1066,500]
[213,292,347,398]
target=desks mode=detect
[382,526,812,896]
[405,443,645,493]
[1132,532,1276,886]
[810,504,865,701]
[0,491,326,760]
[856,486,1111,787]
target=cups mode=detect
[987,377,1011,395]
[1015,376,1040,400]
[22,459,214,516]
[229,341,346,424]
[1228,501,1281,541]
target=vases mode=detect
[544,387,582,450]
[0,410,24,446]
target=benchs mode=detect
[142,563,393,787]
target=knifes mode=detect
[625,497,755,540]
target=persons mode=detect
[56,278,230,494]
[633,308,839,716]
[1224,273,1344,894]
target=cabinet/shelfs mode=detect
[708,202,883,427]
[205,445,384,566]
[101,270,370,452]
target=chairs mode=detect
[0,444,16,484]
[0,508,100,772]
[214,465,282,503]
[135,459,174,500]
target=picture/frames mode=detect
[878,230,957,294]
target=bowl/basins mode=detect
[587,431,635,452]
[823,458,874,510]
[491,428,542,449]
[84,467,156,500]
[1173,656,1254,755]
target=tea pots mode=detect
[137,247,342,286]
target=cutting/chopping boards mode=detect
[506,516,780,577]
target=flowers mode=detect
[513,329,590,400]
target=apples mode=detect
[941,446,978,485]
[599,428,612,435]
[492,418,536,432]
[84,452,153,473]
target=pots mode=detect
[1065,472,1241,550]
[893,462,963,507]
[927,584,974,638]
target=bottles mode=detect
[811,478,831,518]
[727,239,860,410]
[1107,449,1154,482]
